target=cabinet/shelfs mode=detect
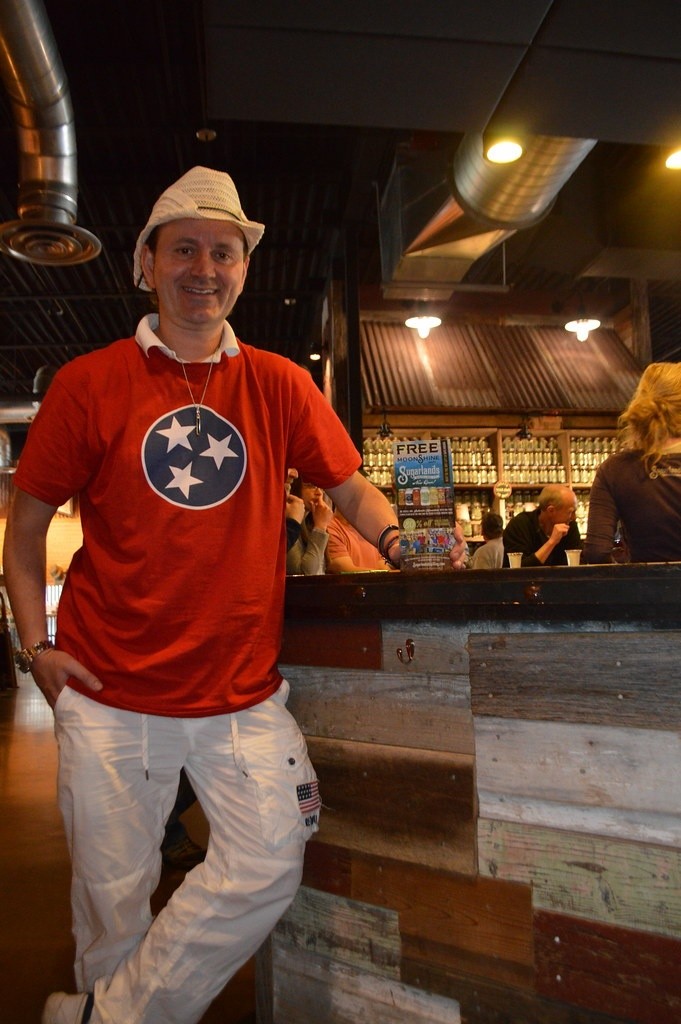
[363,426,625,553]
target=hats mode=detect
[134,166,265,291]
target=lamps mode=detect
[378,413,394,438]
[516,415,531,440]
[405,294,441,339]
[552,291,602,342]
[483,133,527,164]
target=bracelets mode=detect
[385,535,401,566]
[378,523,400,556]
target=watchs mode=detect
[14,640,54,674]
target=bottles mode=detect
[570,437,621,483]
[456,491,490,536]
[506,490,540,524]
[364,437,497,484]
[504,437,566,484]
[573,489,590,534]
[383,490,396,514]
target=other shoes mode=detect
[42,992,94,1024]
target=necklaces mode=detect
[155,329,219,435]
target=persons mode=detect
[502,484,581,568]
[401,532,452,554]
[580,362,681,565]
[285,467,395,575]
[2,165,466,1024]
[469,512,504,569]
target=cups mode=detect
[508,552,523,568]
[564,550,582,566]
